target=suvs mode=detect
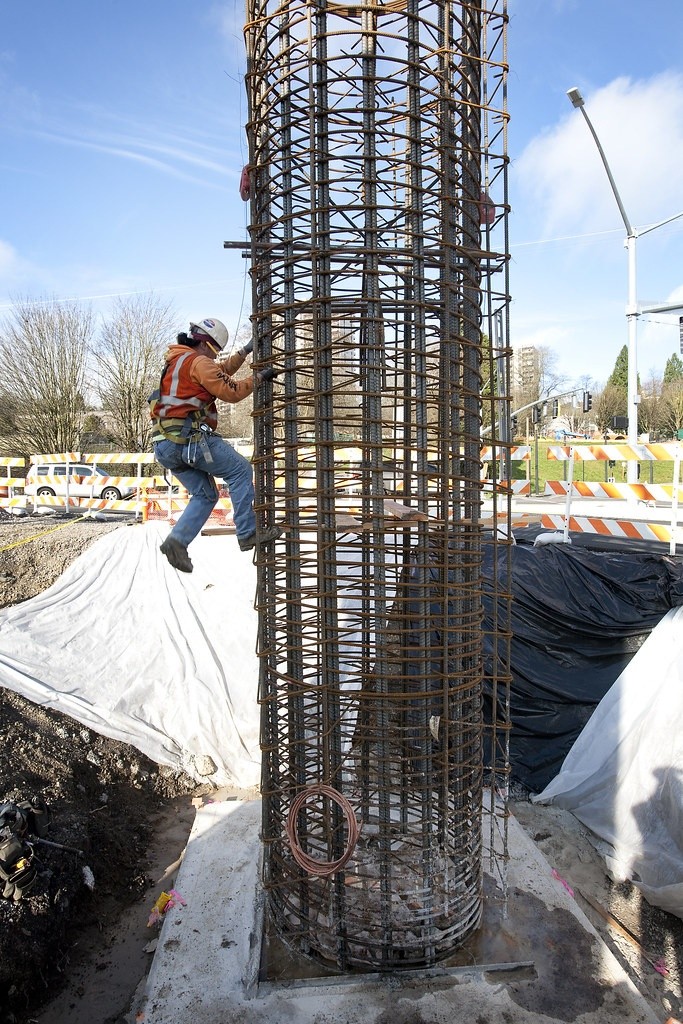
[23,463,134,501]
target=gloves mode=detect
[259,368,279,381]
[244,338,263,354]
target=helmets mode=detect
[190,318,228,351]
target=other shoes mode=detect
[160,535,193,572]
[238,525,283,551]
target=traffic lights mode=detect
[582,389,593,411]
[532,407,542,423]
[510,415,518,430]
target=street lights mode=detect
[565,86,683,484]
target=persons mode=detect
[148,319,281,573]
